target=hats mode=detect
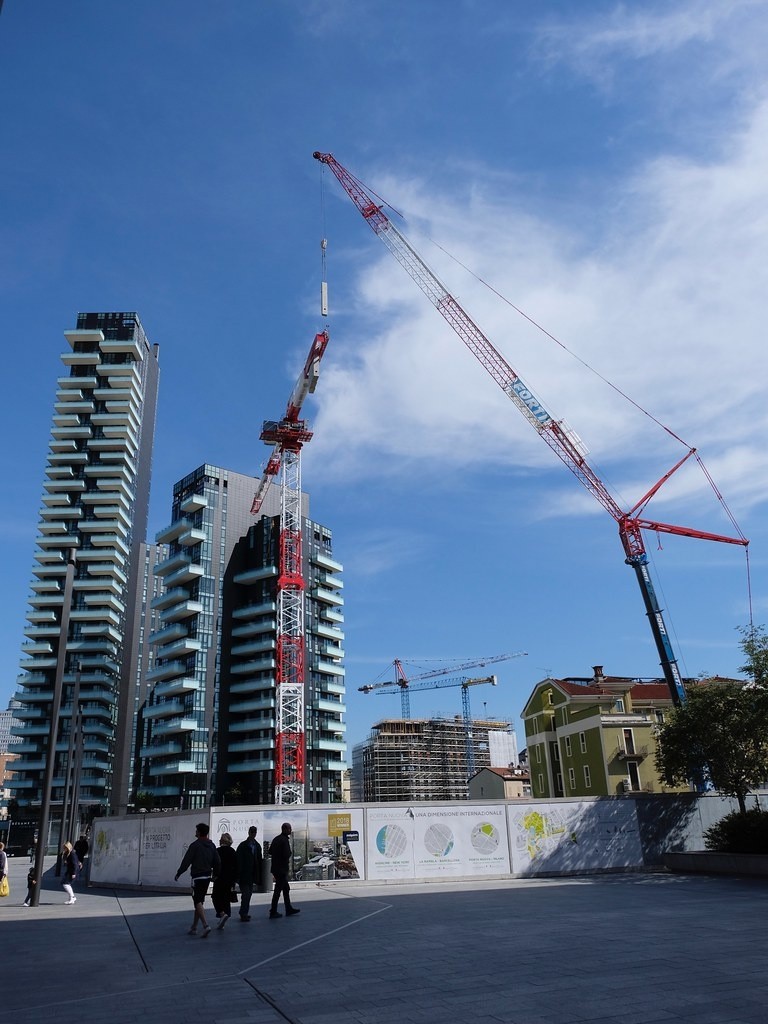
[196,824,210,833]
[223,834,233,845]
[29,867,34,872]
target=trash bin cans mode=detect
[258,857,274,894]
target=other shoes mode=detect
[269,911,283,919]
[202,927,211,937]
[241,914,251,922]
[23,903,28,906]
[285,908,301,916]
[65,897,77,904]
[187,928,197,934]
[217,914,228,929]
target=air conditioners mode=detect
[622,778,631,785]
[624,785,632,791]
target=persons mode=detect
[211,833,236,930]
[236,826,263,921]
[174,823,221,937]
[268,823,301,919]
[0,836,89,907]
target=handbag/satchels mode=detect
[0,876,9,897]
[78,861,82,870]
[230,891,239,903]
